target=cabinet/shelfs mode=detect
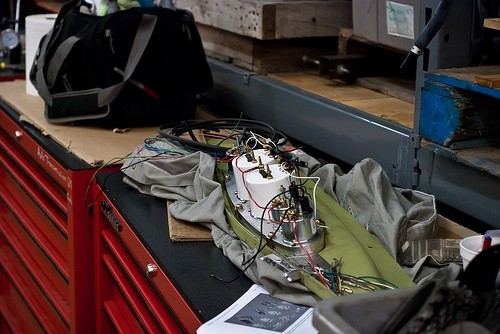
[0,76,251,334]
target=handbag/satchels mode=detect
[29,1,214,127]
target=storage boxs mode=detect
[173,1,419,71]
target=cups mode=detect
[460,234,500,270]
